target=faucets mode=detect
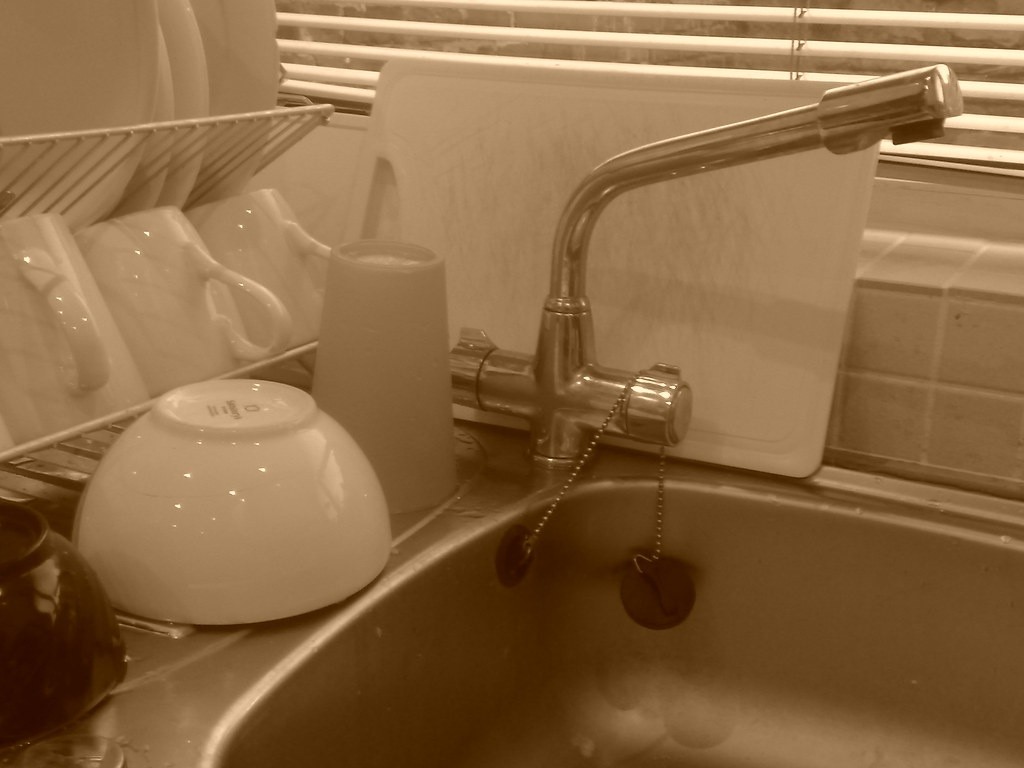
[448,62,965,467]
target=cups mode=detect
[1,212,145,447]
[67,203,294,401]
[306,233,456,519]
[180,187,334,354]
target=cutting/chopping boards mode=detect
[332,52,892,477]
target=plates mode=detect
[0,0,279,224]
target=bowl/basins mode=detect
[0,497,130,757]
[73,377,394,626]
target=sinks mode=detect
[199,472,1023,768]
